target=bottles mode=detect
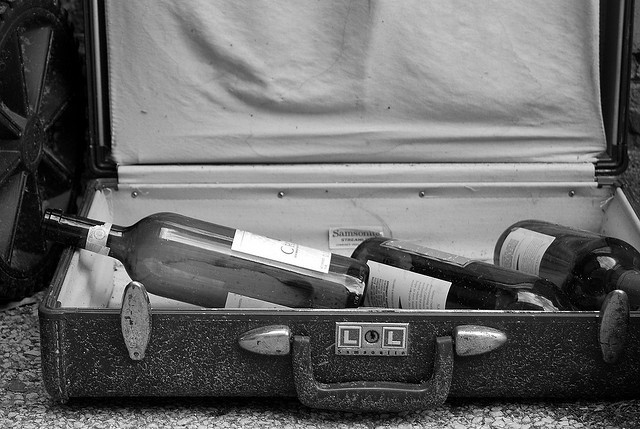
[42,206,370,308]
[493,220,639,310]
[347,238,573,311]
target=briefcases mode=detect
[36,0,640,413]
